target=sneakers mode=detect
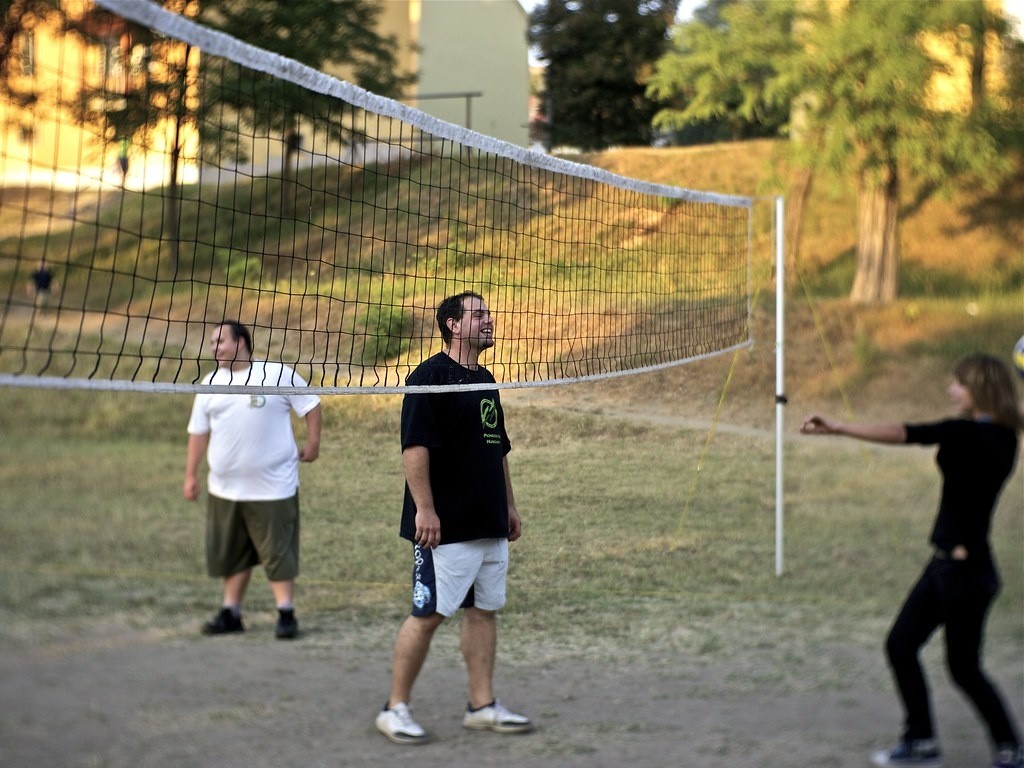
[986,747,1024,768]
[871,738,941,767]
[463,698,530,734]
[374,700,427,744]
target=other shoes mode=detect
[275,608,297,638]
[202,609,244,634]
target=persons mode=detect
[28,260,59,318]
[800,355,1024,767]
[185,320,325,639]
[376,291,531,748]
